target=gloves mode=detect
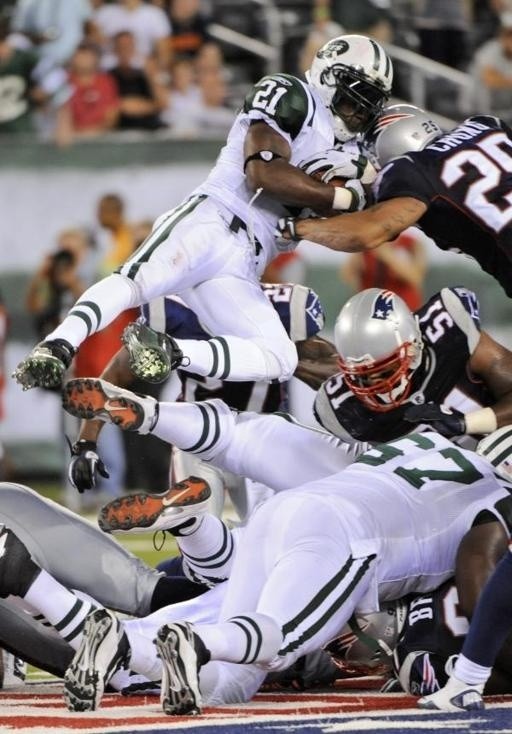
[389,642,449,699]
[344,176,368,211]
[273,215,300,242]
[62,433,109,496]
[401,404,465,439]
[294,148,379,186]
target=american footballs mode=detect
[300,167,347,189]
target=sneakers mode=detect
[416,675,489,713]
[118,321,192,387]
[63,608,132,713]
[98,473,213,537]
[10,338,77,393]
[0,524,31,601]
[58,377,160,438]
[151,618,213,720]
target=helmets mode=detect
[358,103,444,176]
[333,285,425,416]
[307,34,395,145]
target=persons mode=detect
[12,34,394,393]
[0,0,238,136]
[69,282,342,526]
[415,539,512,713]
[0,482,339,693]
[63,424,512,717]
[59,375,364,583]
[29,194,151,335]
[276,104,512,298]
[330,575,511,694]
[0,525,228,696]
[312,286,511,443]
[240,1,512,94]
